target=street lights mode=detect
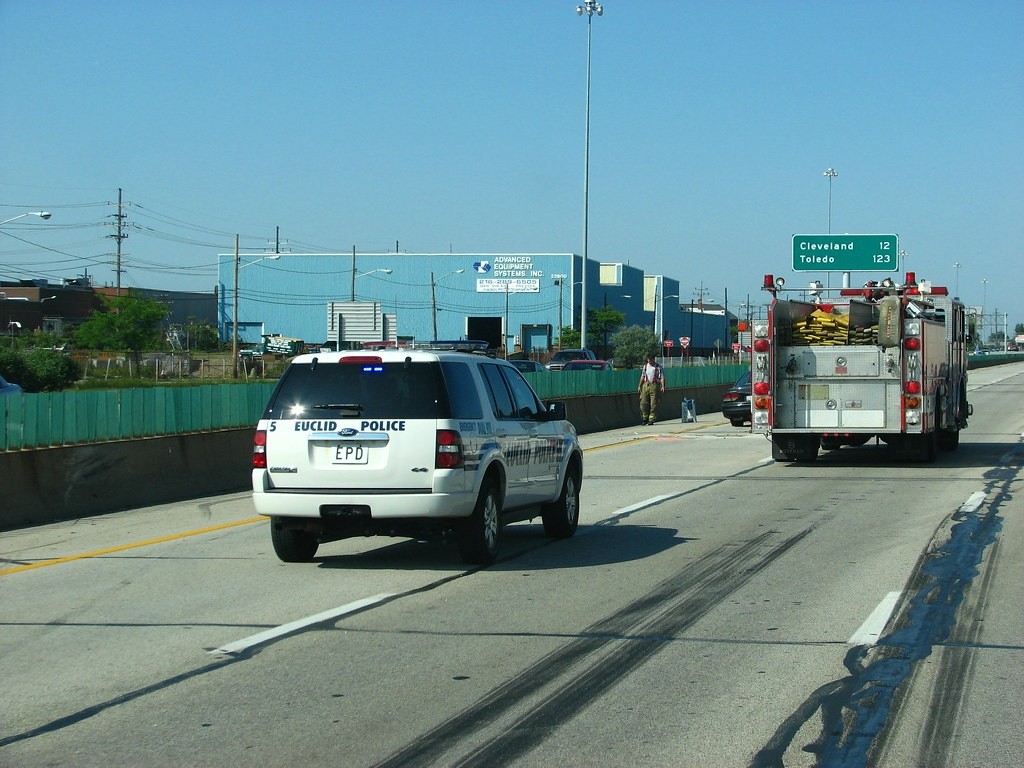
[232,233,282,377]
[430,269,465,341]
[899,249,908,285]
[558,277,583,347]
[981,278,989,313]
[505,282,538,356]
[822,168,838,297]
[351,244,393,303]
[953,262,962,296]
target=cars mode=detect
[0,376,23,394]
[721,370,752,426]
[508,347,613,373]
[973,342,1018,355]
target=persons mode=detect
[637,354,665,426]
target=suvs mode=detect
[251,339,584,566]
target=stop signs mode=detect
[732,343,740,350]
[680,337,689,346]
[664,340,673,348]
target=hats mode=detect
[646,351,657,358]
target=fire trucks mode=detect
[750,271,973,465]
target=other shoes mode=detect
[648,421,654,425]
[642,420,647,426]
